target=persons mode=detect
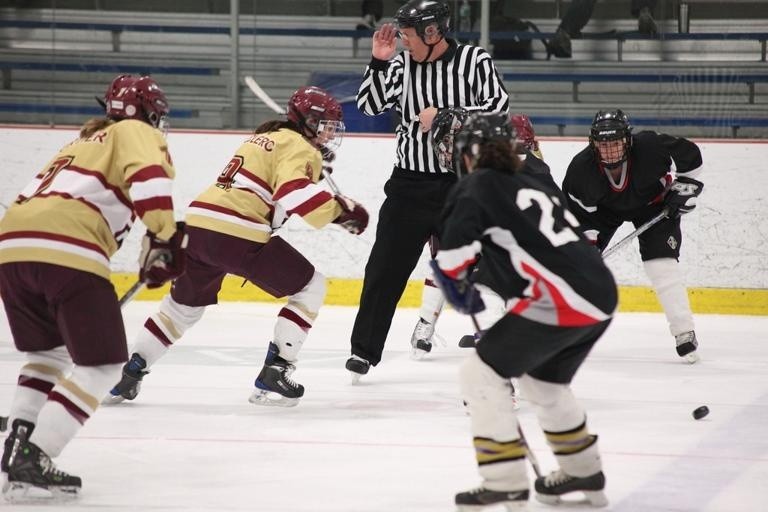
[428,118,615,505]
[558,109,703,358]
[409,111,542,353]
[110,85,368,404]
[1,74,180,491]
[346,1,657,57]
[344,1,510,373]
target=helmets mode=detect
[393,0,451,36]
[103,74,170,128]
[431,107,539,175]
[589,108,635,170]
[286,86,346,160]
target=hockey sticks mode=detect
[459,211,668,348]
[0,255,167,431]
[244,77,339,194]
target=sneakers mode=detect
[356,14,376,30]
[116,353,149,400]
[675,330,698,356]
[255,341,304,398]
[535,469,604,494]
[456,487,529,504]
[1,418,81,493]
[638,6,658,35]
[345,354,371,374]
[411,317,435,352]
[548,27,572,57]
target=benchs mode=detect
[0,1,766,142]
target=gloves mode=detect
[661,176,704,221]
[139,222,190,289]
[429,261,485,314]
[333,193,369,235]
